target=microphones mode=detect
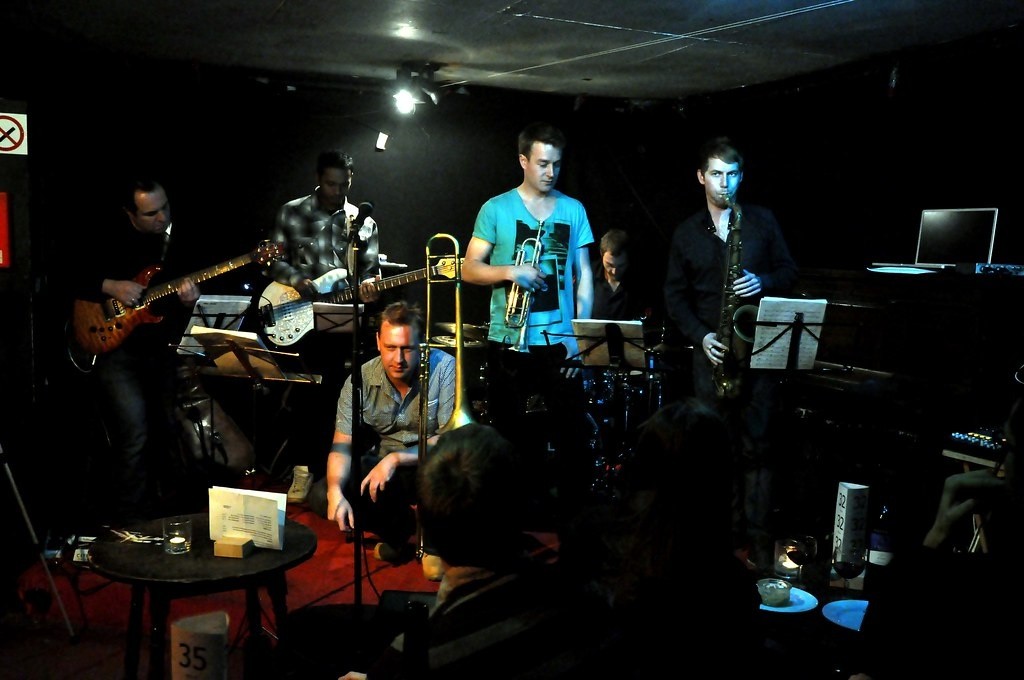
[346,202,374,244]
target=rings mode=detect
[708,345,713,350]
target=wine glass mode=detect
[833,545,868,594]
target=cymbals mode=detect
[430,334,488,348]
[435,320,488,342]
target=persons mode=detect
[306,301,476,562]
[662,143,799,519]
[590,228,645,322]
[337,425,603,680]
[257,146,381,327]
[86,175,203,527]
[848,399,1023,679]
[460,127,596,441]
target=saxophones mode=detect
[707,193,760,401]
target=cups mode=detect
[163,515,193,555]
[773,538,803,580]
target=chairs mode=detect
[406,536,608,680]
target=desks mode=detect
[86,513,317,680]
[739,542,902,650]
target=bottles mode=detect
[869,506,890,576]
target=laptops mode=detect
[872,208,998,269]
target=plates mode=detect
[759,587,818,613]
[822,599,870,631]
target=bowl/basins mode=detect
[757,578,792,607]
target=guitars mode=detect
[69,237,286,357]
[257,255,467,348]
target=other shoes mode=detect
[421,549,444,582]
[373,542,394,561]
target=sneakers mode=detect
[287,466,312,502]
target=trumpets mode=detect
[502,218,546,356]
